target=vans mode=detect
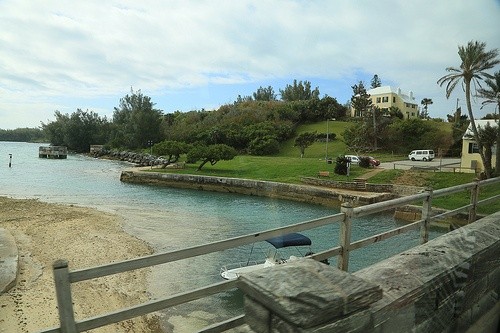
[408,149,434,162]
[345,154,361,163]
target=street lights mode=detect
[326,118,336,164]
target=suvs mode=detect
[363,156,380,166]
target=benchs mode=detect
[319,171,331,178]
